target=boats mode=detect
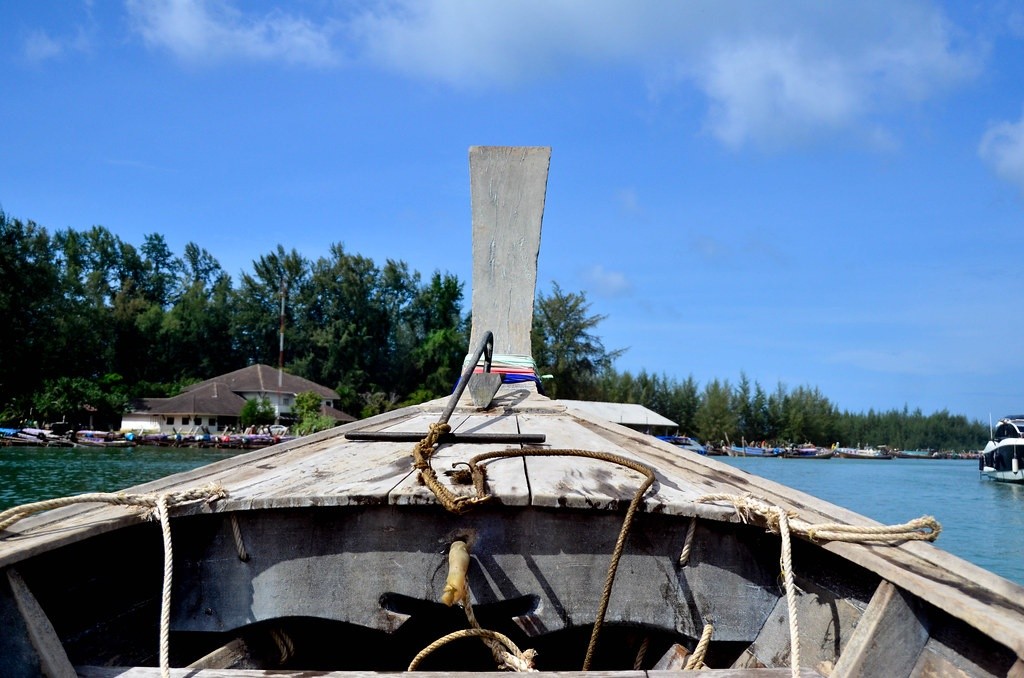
[655,437,707,455]
[731,441,892,459]
[0,425,298,448]
[894,446,980,459]
[977,412,1024,485]
